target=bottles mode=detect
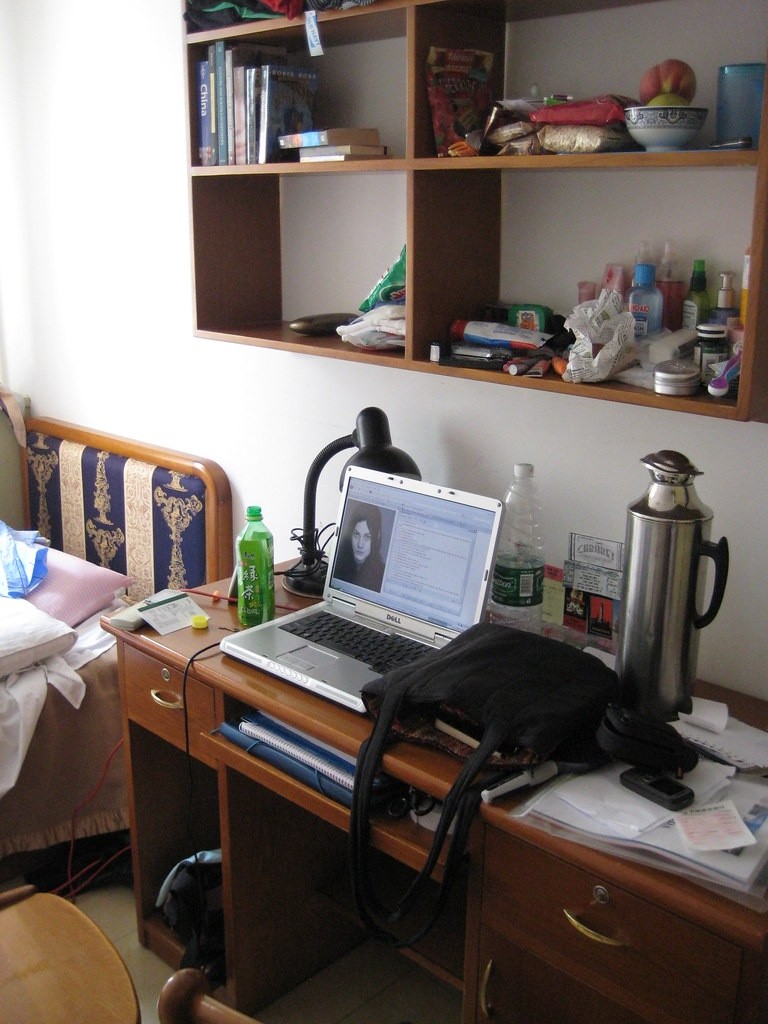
[711,273,739,328]
[489,463,544,636]
[236,506,276,627]
[654,243,685,332]
[683,260,709,328]
[729,327,745,379]
[693,325,729,389]
[625,264,663,343]
[430,342,442,362]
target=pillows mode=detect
[0,597,80,677]
[28,548,133,629]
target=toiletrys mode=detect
[706,270,740,327]
[656,238,687,332]
[449,319,555,350]
[680,256,711,332]
[576,281,598,306]
[624,240,661,305]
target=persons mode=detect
[334,503,386,593]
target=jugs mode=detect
[616,450,729,722]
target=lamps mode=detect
[283,406,422,597]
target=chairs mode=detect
[0,884,143,1024]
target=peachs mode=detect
[640,58,696,101]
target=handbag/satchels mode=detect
[161,861,225,992]
[342,621,619,931]
[596,701,699,775]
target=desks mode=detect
[99,555,768,1024]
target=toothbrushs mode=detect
[706,342,746,398]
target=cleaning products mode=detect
[335,242,408,351]
[623,263,664,339]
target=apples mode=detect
[645,93,691,107]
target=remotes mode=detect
[110,589,181,631]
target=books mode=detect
[198,40,323,166]
[238,713,393,794]
[277,126,388,162]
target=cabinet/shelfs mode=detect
[178,0,768,424]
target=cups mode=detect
[717,63,765,150]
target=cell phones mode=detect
[620,768,695,811]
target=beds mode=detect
[0,416,231,862]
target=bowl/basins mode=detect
[624,107,709,152]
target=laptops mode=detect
[219,464,505,717]
